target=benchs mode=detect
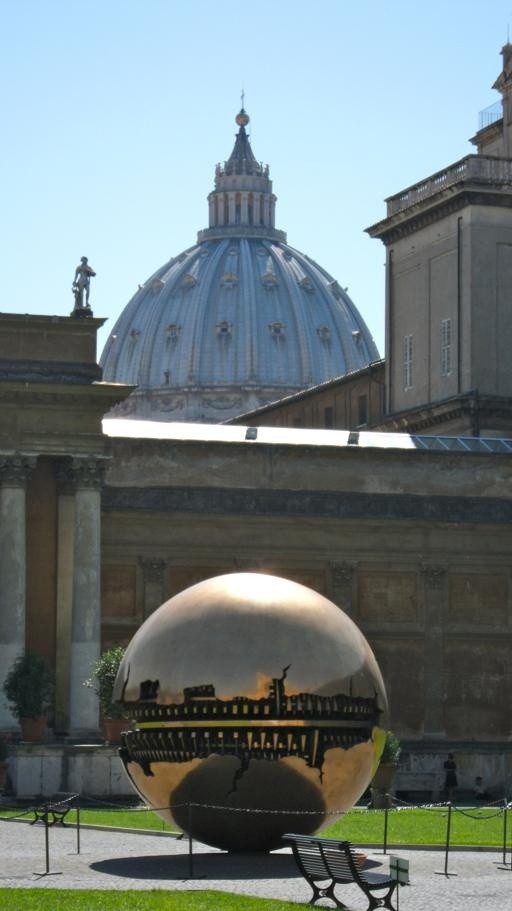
[280,831,409,910]
[30,791,79,827]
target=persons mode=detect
[74,257,96,308]
